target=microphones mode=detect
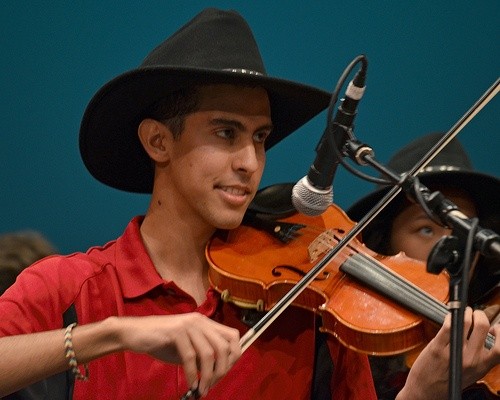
[292,68,367,216]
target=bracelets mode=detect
[64,322,90,381]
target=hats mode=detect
[77,8,338,193]
[345,131,500,309]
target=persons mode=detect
[0,131,500,400]
[0,7,500,400]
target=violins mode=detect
[204,182,496,357]
[405,272,500,398]
